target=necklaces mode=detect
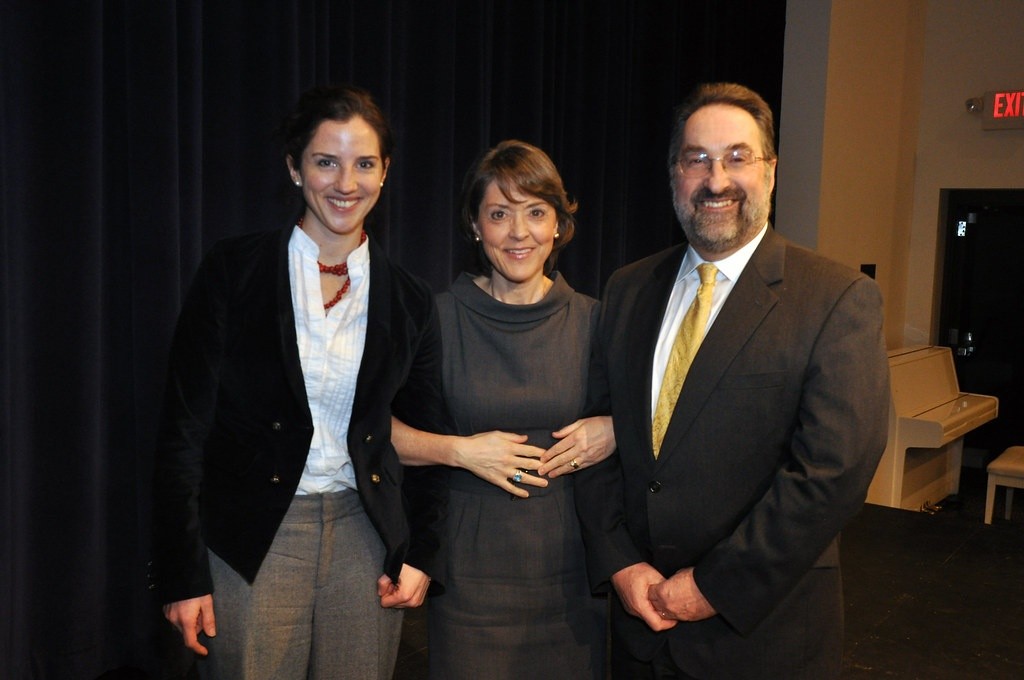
[298,219,366,309]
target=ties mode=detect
[652,263,720,458]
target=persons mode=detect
[391,139,617,680]
[581,83,890,680]
[150,89,443,680]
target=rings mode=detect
[514,470,522,481]
[660,612,665,618]
[571,461,579,469]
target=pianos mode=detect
[865,342,1000,518]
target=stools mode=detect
[985,446,1024,523]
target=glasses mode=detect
[671,149,772,178]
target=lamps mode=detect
[965,89,1024,130]
